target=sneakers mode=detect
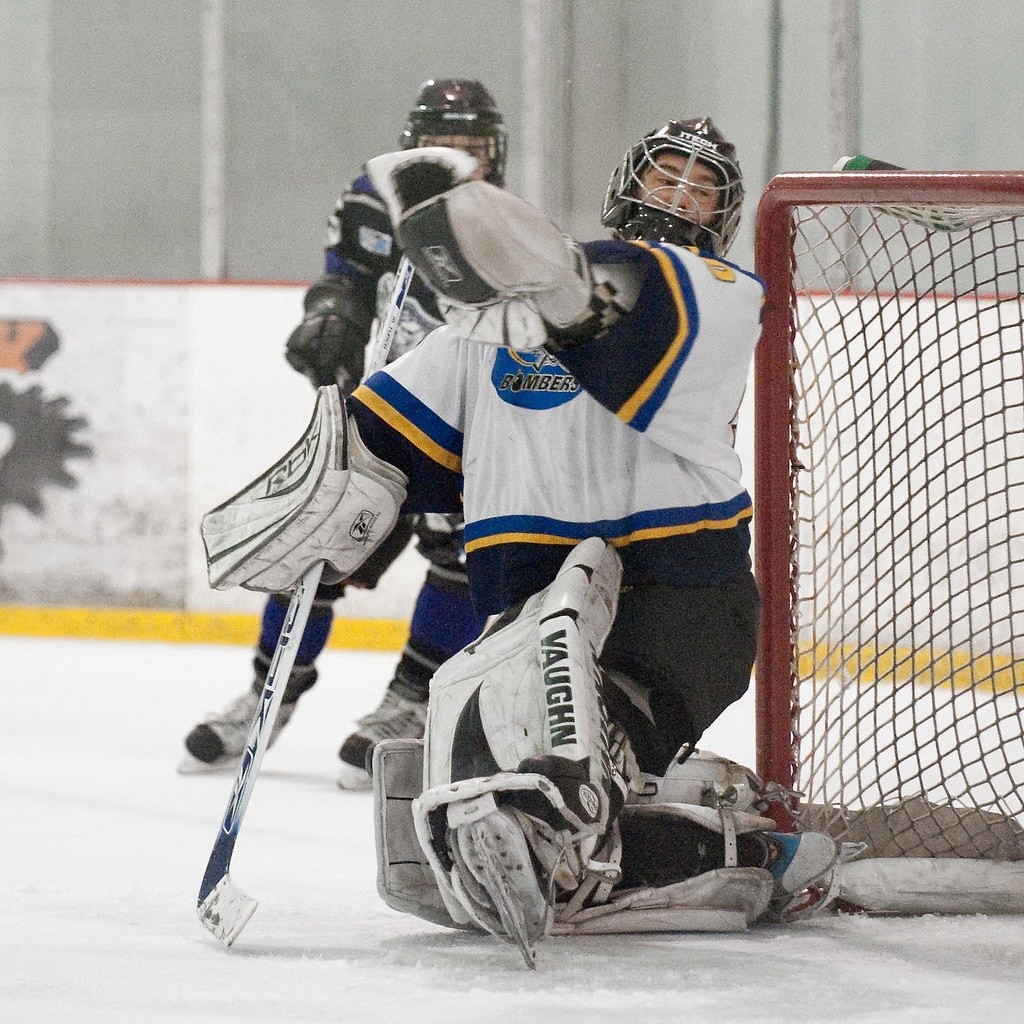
[180,646,318,772]
[701,760,784,815]
[751,832,868,923]
[335,647,439,789]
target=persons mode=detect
[185,78,508,767]
[201,117,867,937]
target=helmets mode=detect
[399,79,507,187]
[600,116,747,257]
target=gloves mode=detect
[287,272,374,390]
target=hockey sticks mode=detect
[194,252,417,949]
